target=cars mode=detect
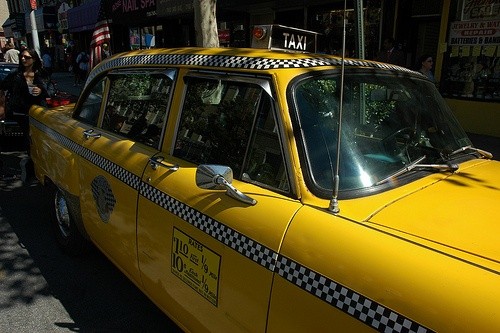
[25,21,500,332]
[1,69,87,139]
[0,62,19,84]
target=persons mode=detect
[450,56,482,95]
[381,38,402,65]
[417,55,434,80]
[0,42,113,187]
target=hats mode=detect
[8,43,15,49]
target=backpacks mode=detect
[80,53,89,63]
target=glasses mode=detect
[428,60,433,63]
[20,55,33,60]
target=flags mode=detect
[88,0,110,74]
